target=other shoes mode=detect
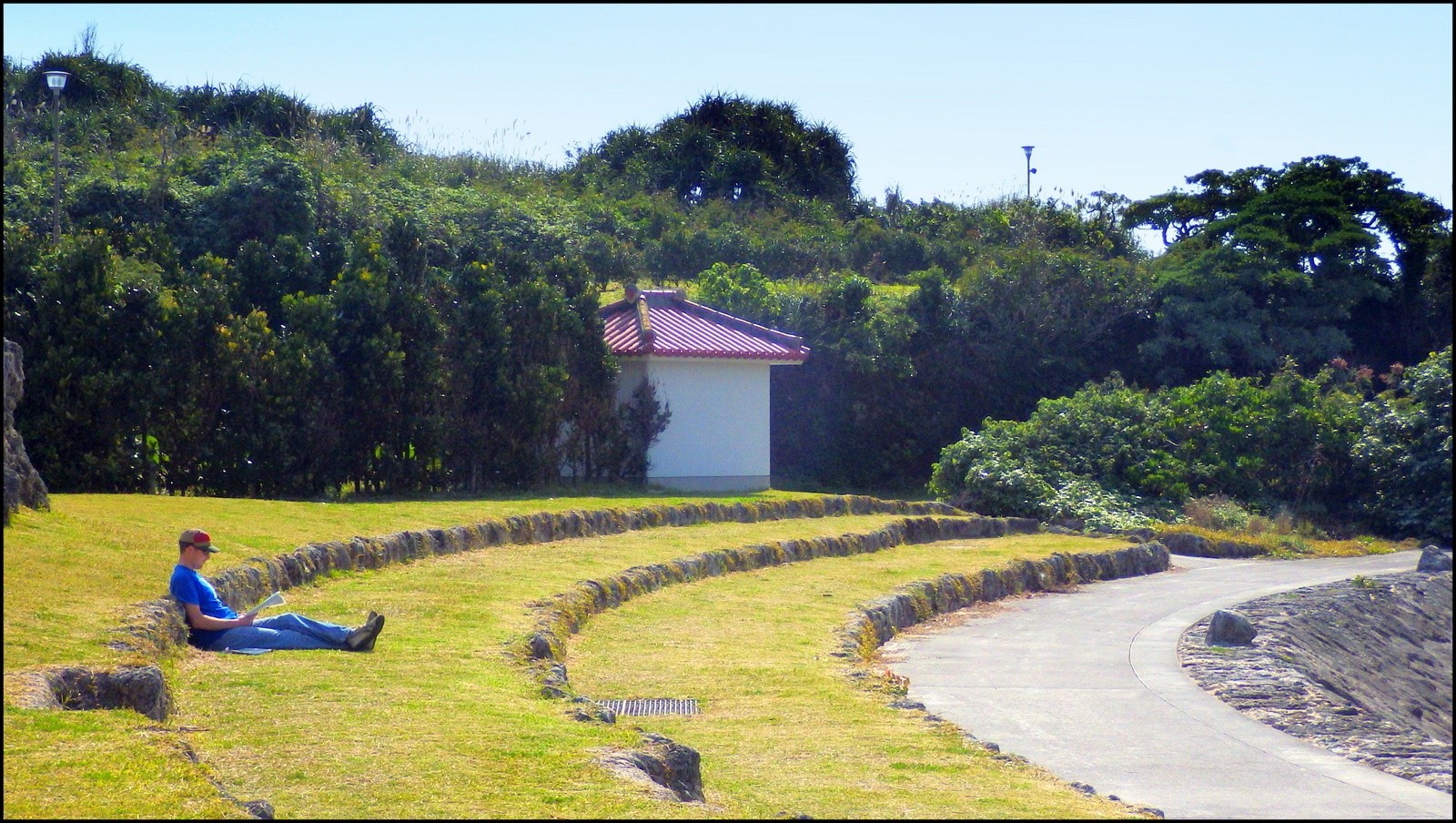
[347,615,384,651]
[361,611,377,650]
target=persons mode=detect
[170,529,385,651]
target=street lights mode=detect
[1021,147,1037,196]
[43,72,71,244]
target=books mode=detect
[232,648,273,655]
[244,590,285,618]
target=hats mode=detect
[179,529,220,553]
